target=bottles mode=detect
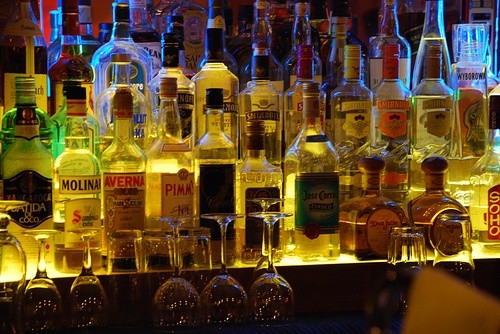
[0,1,500,277]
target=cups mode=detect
[432,212,476,291]
[384,225,429,317]
[105,227,211,331]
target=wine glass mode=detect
[64,226,108,330]
[16,230,64,333]
[197,213,247,328]
[247,211,295,328]
[245,196,285,288]
[151,215,208,327]
[0,199,30,334]
